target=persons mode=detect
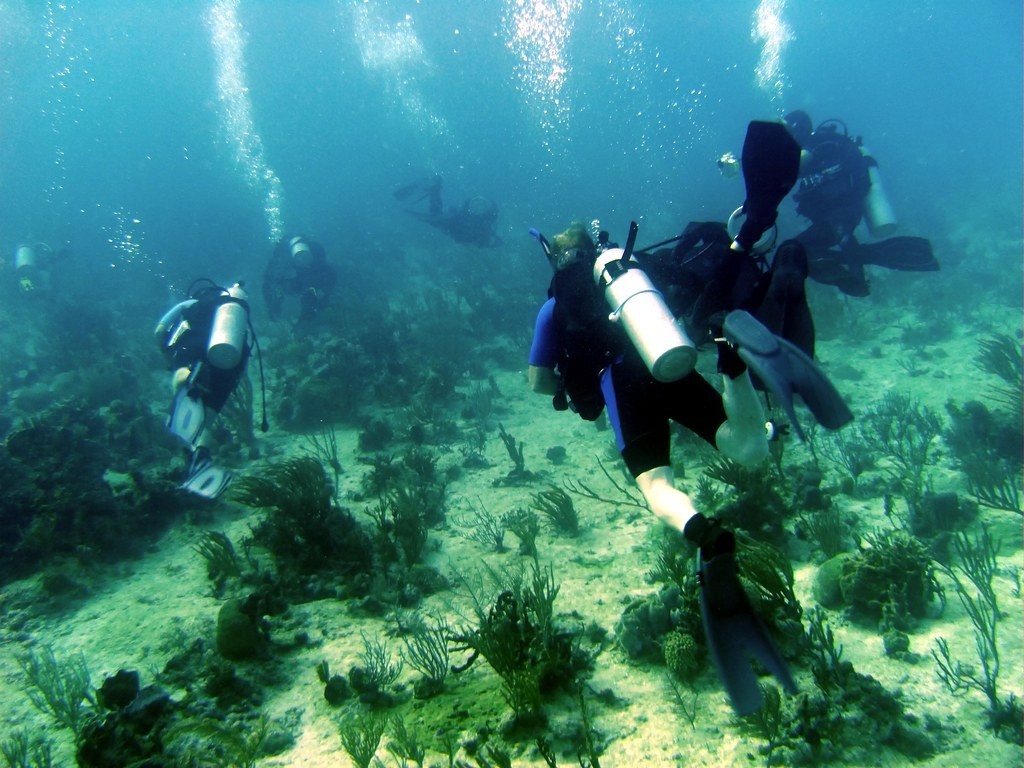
[155,288,246,502]
[716,112,941,297]
[528,223,853,716]
[264,234,333,314]
[403,176,502,248]
